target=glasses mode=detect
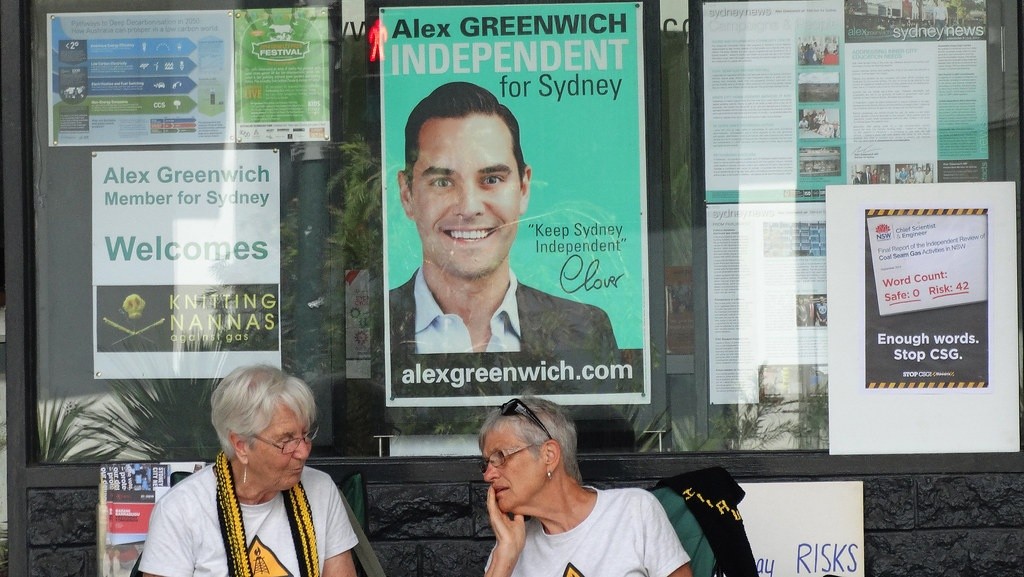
[253,426,320,453]
[478,443,544,474]
[498,398,553,440]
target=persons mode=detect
[478,397,693,577]
[389,80,624,398]
[895,163,933,184]
[800,41,840,64]
[138,365,359,577]
[853,165,886,184]
[799,109,840,138]
